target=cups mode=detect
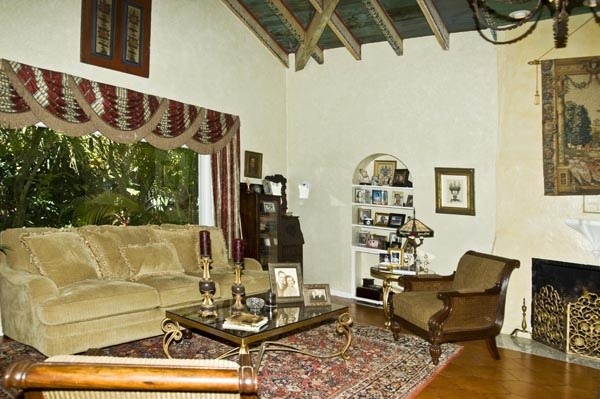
[265,307,277,323]
[265,292,276,307]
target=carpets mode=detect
[0,319,465,399]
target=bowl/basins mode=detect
[247,311,264,316]
[246,297,264,311]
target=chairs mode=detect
[388,250,521,365]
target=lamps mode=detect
[395,207,434,271]
[466,0,600,49]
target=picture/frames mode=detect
[356,189,371,203]
[372,190,388,205]
[387,248,404,266]
[268,262,304,304]
[374,212,390,227]
[391,169,412,187]
[435,167,475,216]
[374,160,396,184]
[389,232,402,247]
[391,191,404,206]
[301,283,333,307]
[357,232,370,246]
[358,207,371,225]
[379,252,390,270]
[374,234,387,249]
[244,150,262,179]
[405,195,413,207]
[391,241,401,248]
[388,213,405,228]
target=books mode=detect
[222,311,268,334]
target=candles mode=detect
[233,239,244,262]
[200,230,211,257]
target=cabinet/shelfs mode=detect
[240,183,280,271]
[352,184,414,257]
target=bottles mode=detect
[423,253,429,272]
[415,256,420,272]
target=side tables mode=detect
[370,265,436,329]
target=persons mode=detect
[276,271,286,297]
[283,275,297,297]
[310,291,324,300]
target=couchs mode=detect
[0,223,270,359]
